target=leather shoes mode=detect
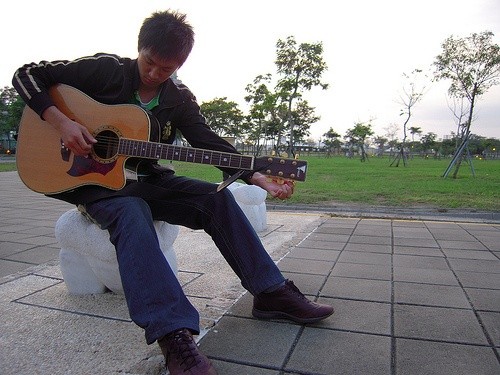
[157,328,218,375]
[252,279,335,324]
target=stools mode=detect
[56,208,178,294]
[215,181,266,233]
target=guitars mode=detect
[16,82,313,196]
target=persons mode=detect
[12,9,335,375]
